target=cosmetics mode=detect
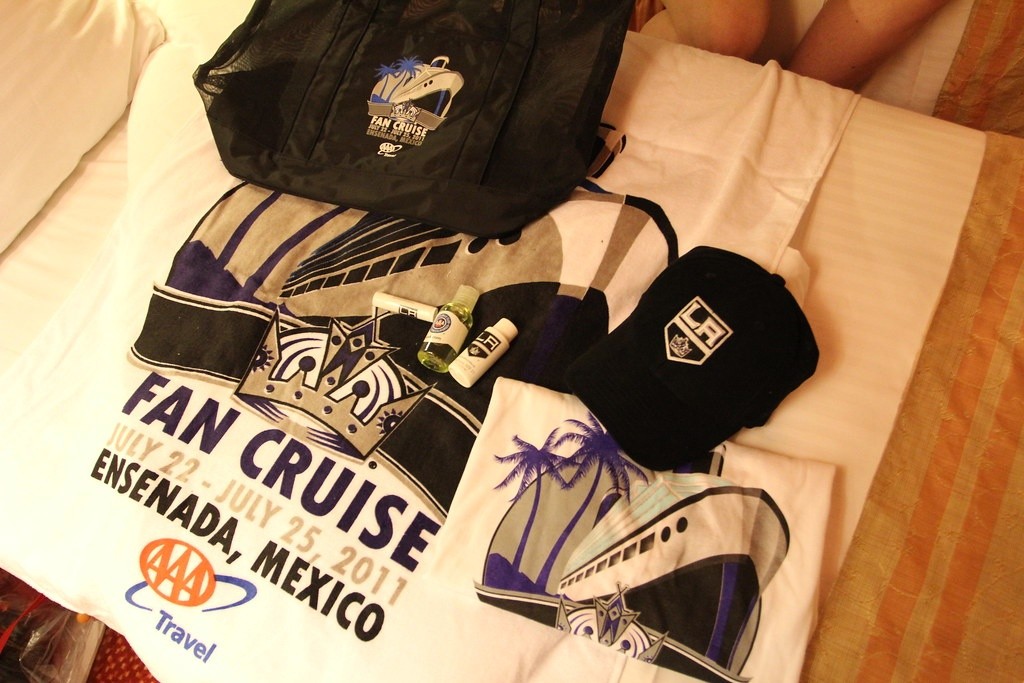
[372,276,518,388]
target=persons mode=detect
[640,0,956,90]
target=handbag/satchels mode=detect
[192,0,636,239]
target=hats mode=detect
[563,246,820,472]
